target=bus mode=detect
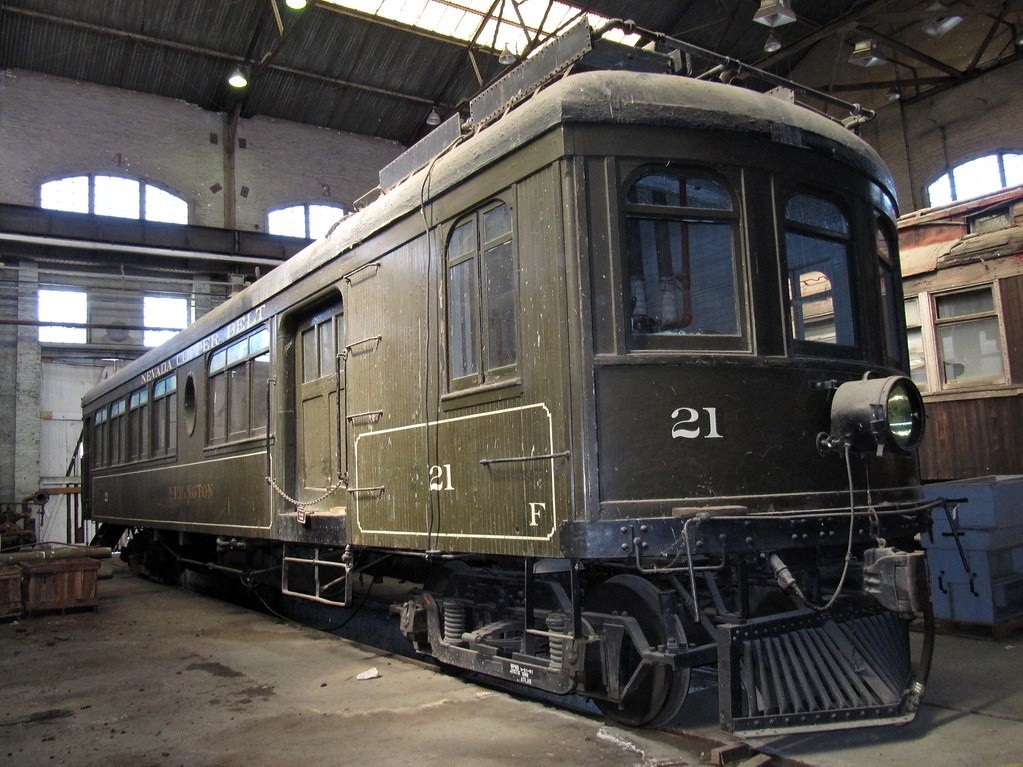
[69,12,943,736]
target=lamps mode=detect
[753,0,797,28]
[764,30,781,52]
[426,106,441,125]
[848,39,889,67]
[886,87,901,101]
[498,42,515,65]
[229,68,247,89]
[918,1,964,38]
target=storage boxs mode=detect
[0,558,101,618]
[918,474,1023,622]
